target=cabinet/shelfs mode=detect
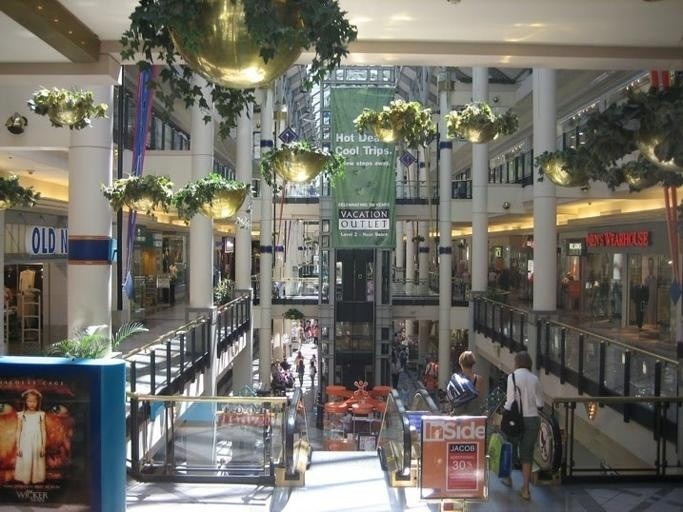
[3,289,42,355]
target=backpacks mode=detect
[501,386,523,444]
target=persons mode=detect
[489,259,514,290]
[13,389,48,485]
[279,350,317,390]
[501,349,544,500]
[454,261,471,280]
[340,410,352,433]
[301,321,318,346]
[643,257,657,325]
[0,378,77,488]
[392,332,408,388]
[451,349,485,416]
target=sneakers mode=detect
[520,488,531,501]
[500,479,512,487]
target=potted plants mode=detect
[174,170,258,223]
[445,101,518,146]
[27,85,109,131]
[213,278,237,326]
[98,175,174,215]
[352,98,439,148]
[531,146,606,188]
[5,108,30,135]
[582,68,682,191]
[121,1,359,141]
[260,135,344,191]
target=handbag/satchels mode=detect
[487,434,512,478]
[446,373,479,408]
